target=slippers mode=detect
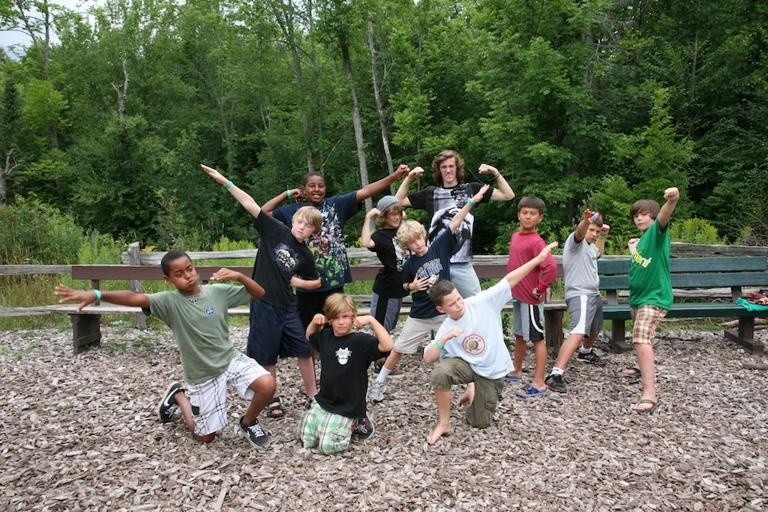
[502,372,522,383]
[621,365,640,376]
[515,382,547,397]
[632,396,663,415]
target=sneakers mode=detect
[158,381,185,423]
[352,411,375,440]
[239,416,271,450]
[577,349,607,366]
[373,357,405,378]
[544,373,567,395]
[372,378,386,404]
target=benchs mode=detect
[594,257,768,341]
[53,265,566,352]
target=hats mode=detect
[377,195,401,212]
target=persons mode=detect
[424,241,558,445]
[298,291,393,456]
[372,184,489,403]
[395,152,515,299]
[261,165,410,329]
[620,187,680,413]
[199,165,323,418]
[360,167,424,376]
[504,194,558,399]
[53,251,275,449]
[545,210,610,393]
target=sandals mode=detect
[305,396,317,410]
[266,398,284,418]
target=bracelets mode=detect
[407,283,411,291]
[225,180,234,188]
[465,198,476,208]
[287,189,292,200]
[95,289,102,303]
[495,172,500,178]
